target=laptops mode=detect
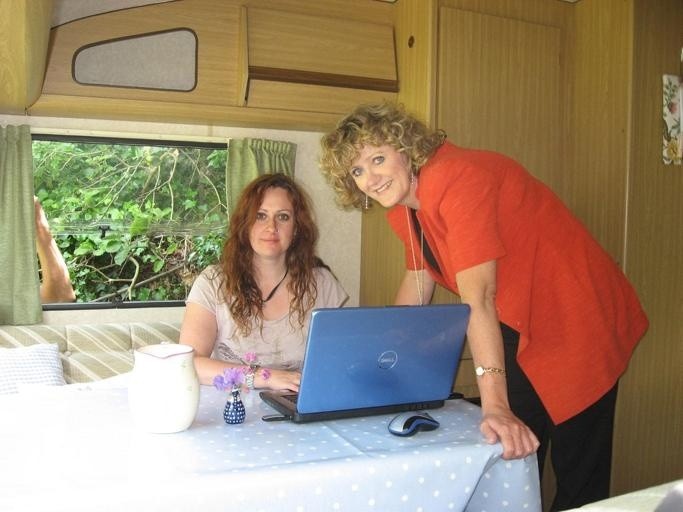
[259,302,470,424]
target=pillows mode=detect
[0,343,66,386]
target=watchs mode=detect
[474,366,506,377]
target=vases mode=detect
[224,391,246,424]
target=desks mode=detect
[0,369,542,512]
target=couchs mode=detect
[0,321,181,384]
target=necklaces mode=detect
[406,206,424,306]
[257,265,289,308]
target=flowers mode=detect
[213,352,270,393]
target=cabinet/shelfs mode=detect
[360,5,566,396]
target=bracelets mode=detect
[246,365,259,390]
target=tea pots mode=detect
[135,341,200,432]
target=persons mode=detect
[316,99,650,511]
[177,172,352,395]
[34,199,77,305]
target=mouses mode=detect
[388,411,440,436]
